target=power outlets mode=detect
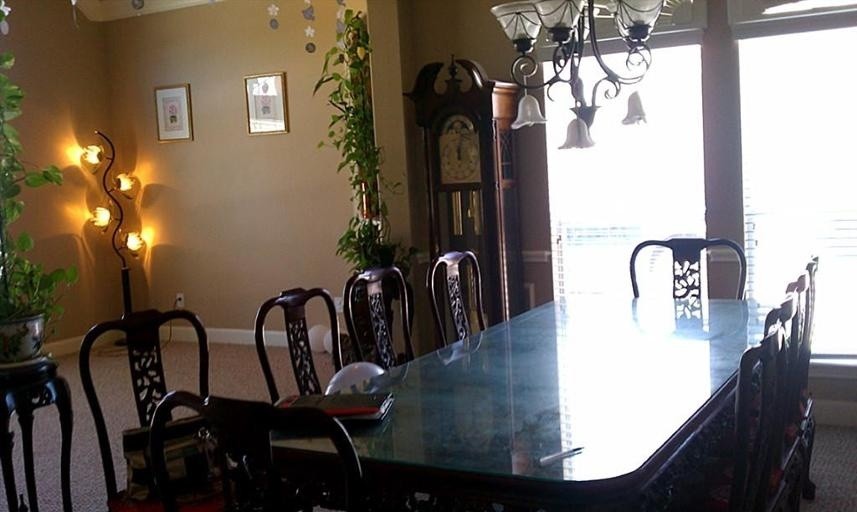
[333,297,344,312]
[177,293,185,308]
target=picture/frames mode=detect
[154,83,192,142]
[242,72,288,137]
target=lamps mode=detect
[490,0,691,152]
[81,131,181,357]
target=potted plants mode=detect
[0,0,81,367]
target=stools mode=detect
[2,349,74,511]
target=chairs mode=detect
[679,329,781,512]
[79,308,210,501]
[806,256,819,497]
[425,250,487,347]
[629,238,747,300]
[779,272,811,512]
[149,389,362,512]
[351,363,409,395]
[435,330,483,368]
[343,267,415,372]
[703,294,802,512]
[632,296,748,340]
[254,287,343,404]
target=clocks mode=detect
[401,52,526,325]
[420,324,531,475]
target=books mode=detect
[272,392,395,421]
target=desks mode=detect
[270,298,805,512]
